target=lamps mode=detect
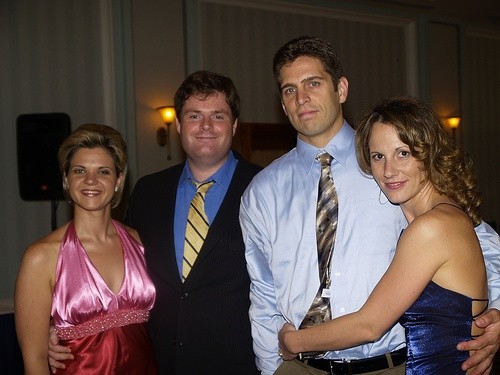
[155,105,176,160]
[447,116,461,148]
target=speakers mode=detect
[16,112,73,200]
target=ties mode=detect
[181,178,216,284]
[297,152,340,327]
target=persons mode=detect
[239,35,500,375]
[14,124,156,375]
[48,71,265,375]
[278,97,489,375]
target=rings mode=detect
[278,353,282,356]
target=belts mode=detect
[296,346,407,375]
